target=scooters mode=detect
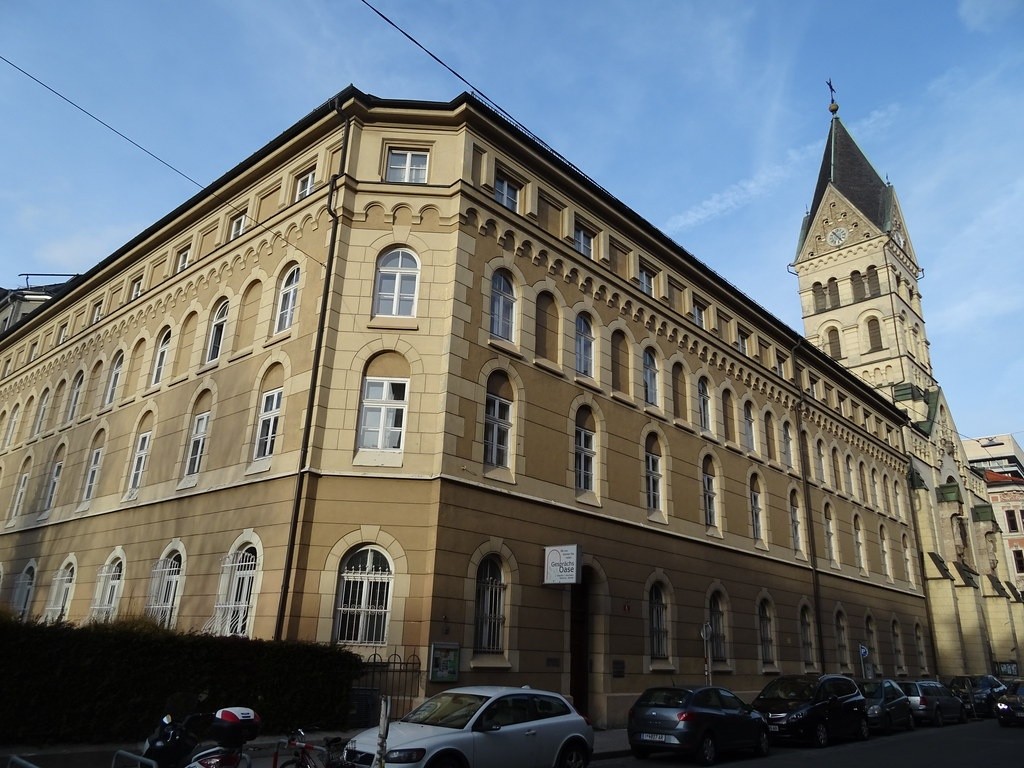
[142,707,261,768]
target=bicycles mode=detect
[279,728,357,768]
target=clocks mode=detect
[895,232,903,247]
[827,228,848,246]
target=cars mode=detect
[627,686,771,766]
[340,685,594,768]
[751,674,869,748]
[895,680,968,727]
[949,674,1024,727]
[854,679,914,736]
